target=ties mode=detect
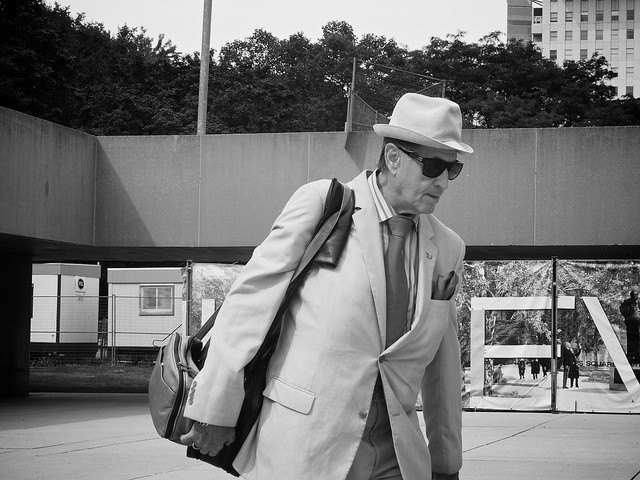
[384,215,412,349]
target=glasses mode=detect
[398,146,463,180]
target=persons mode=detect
[180,92,473,479]
[518,361,525,379]
[531,359,539,379]
[493,367,507,383]
[568,344,582,387]
[620,290,640,321]
[542,361,548,377]
[562,342,573,389]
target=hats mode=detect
[373,93,474,154]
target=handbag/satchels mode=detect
[149,333,199,444]
[186,332,265,478]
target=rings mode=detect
[191,441,201,450]
